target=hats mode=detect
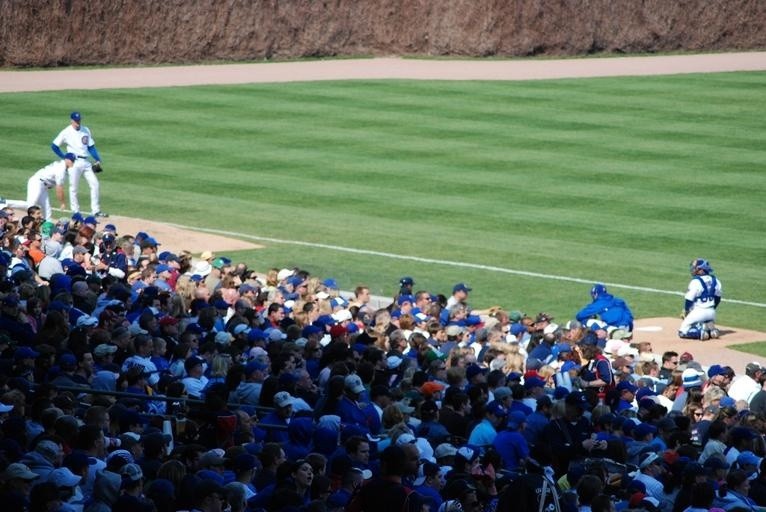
[0,278,766,512]
[64,152,78,161]
[160,250,310,278]
[70,111,81,121]
[0,208,160,277]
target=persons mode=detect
[51,112,109,218]
[24,152,76,222]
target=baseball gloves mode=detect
[92,161,102,173]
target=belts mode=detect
[76,156,88,159]
[40,178,51,190]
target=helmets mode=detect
[690,258,714,276]
